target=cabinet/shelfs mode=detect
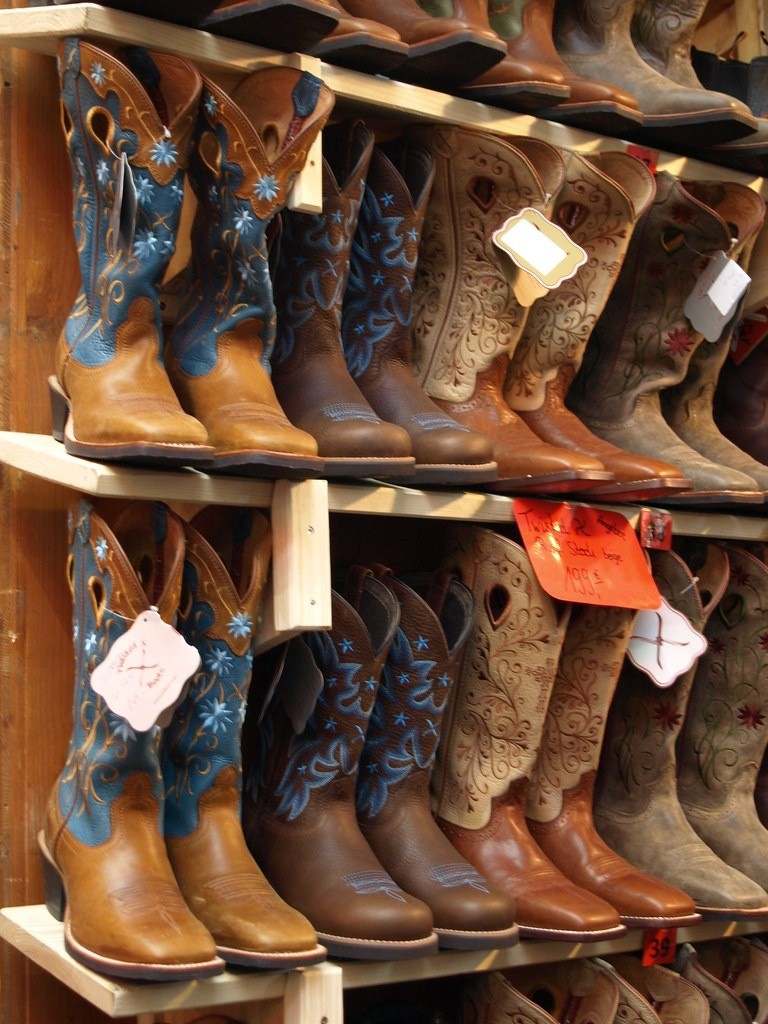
[0,0,768,1024]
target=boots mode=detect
[47,40,768,502]
[120,0,768,166]
[460,935,767,1024]
[37,490,767,978]
